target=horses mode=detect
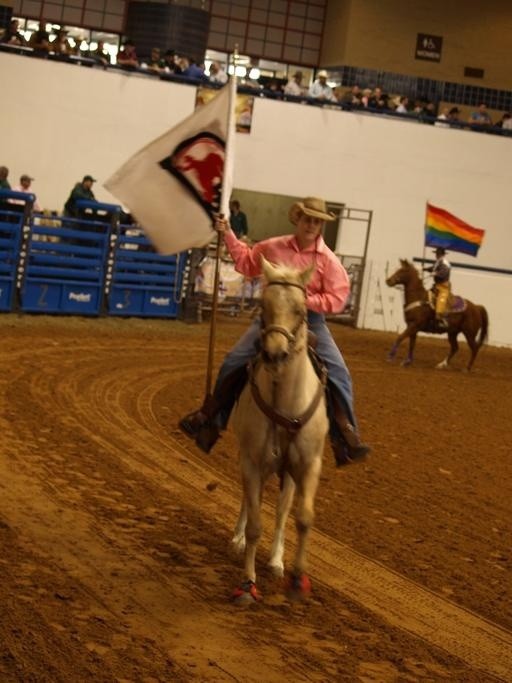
[229,253,330,608]
[386,258,487,375]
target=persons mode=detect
[60,174,98,244]
[420,245,454,326]
[230,198,250,241]
[9,175,42,213]
[177,196,370,467]
[0,165,12,207]
[0,15,511,135]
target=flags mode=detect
[101,70,240,258]
[424,204,485,256]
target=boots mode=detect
[175,394,234,455]
[327,411,372,468]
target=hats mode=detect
[432,247,449,254]
[288,195,337,226]
